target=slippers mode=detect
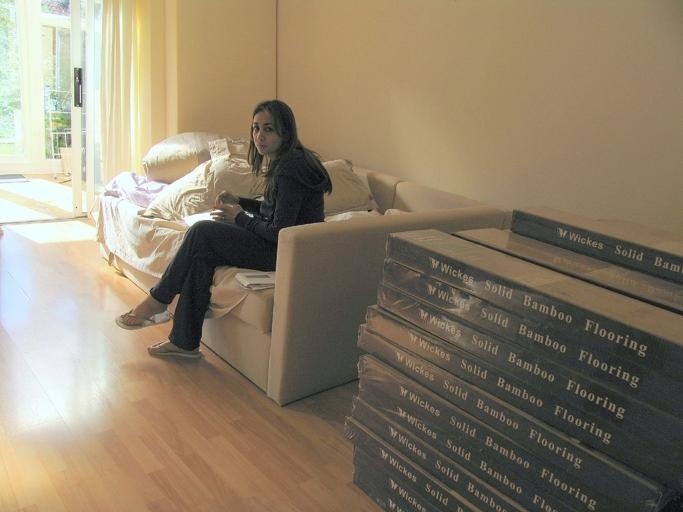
[115,307,171,329]
[147,341,202,359]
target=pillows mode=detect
[320,156,378,214]
[140,132,271,218]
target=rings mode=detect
[221,216,224,220]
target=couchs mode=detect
[99,169,506,406]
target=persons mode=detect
[115,100,332,359]
